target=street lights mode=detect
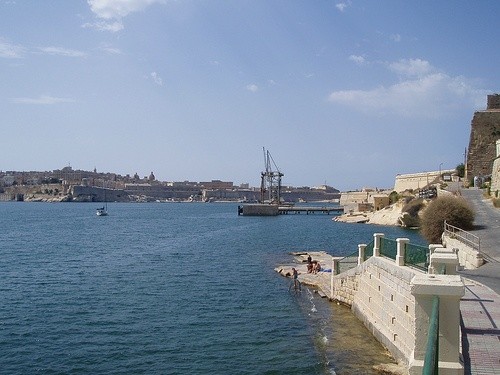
[440,163,444,177]
[427,173,431,186]
[418,180,420,189]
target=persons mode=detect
[312,261,320,274]
[292,268,298,286]
[308,255,311,263]
[307,262,313,273]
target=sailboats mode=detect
[96,187,107,216]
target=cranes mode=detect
[260,146,284,204]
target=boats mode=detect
[24,196,43,202]
